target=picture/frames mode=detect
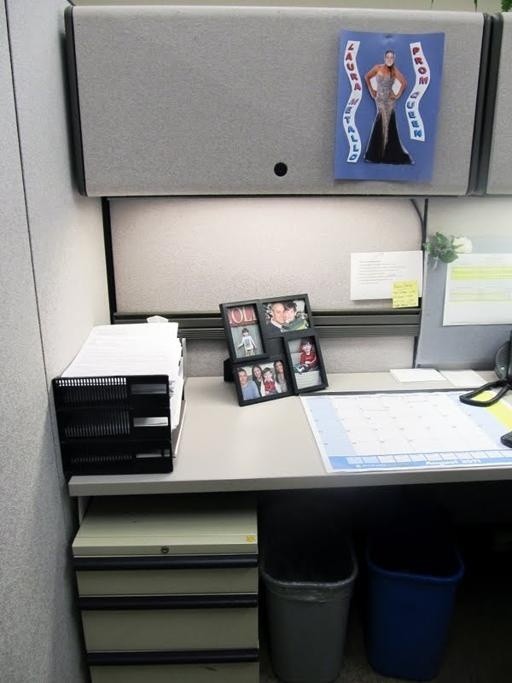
[219,293,329,407]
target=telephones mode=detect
[494,330,512,389]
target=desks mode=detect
[66,369,511,529]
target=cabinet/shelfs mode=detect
[72,496,264,683]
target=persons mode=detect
[265,302,285,334]
[293,336,320,374]
[252,364,266,396]
[237,327,257,357]
[273,360,287,393]
[281,301,309,332]
[362,46,416,164]
[237,368,260,400]
[259,367,282,396]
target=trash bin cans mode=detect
[258,505,465,683]
